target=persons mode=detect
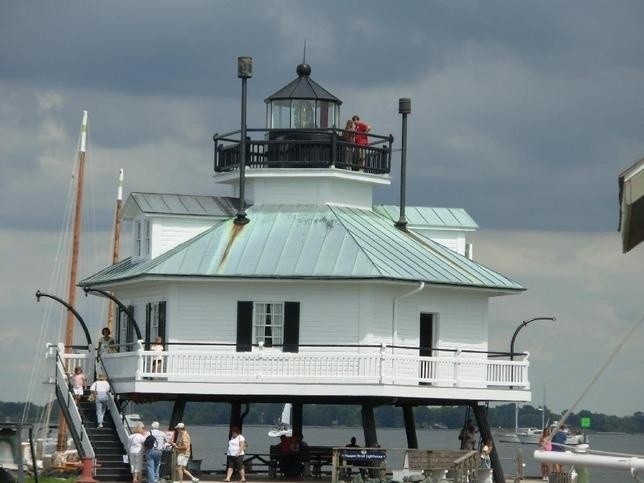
[274,433,291,480]
[343,119,353,169]
[351,114,372,170]
[125,423,147,482]
[291,432,308,480]
[96,326,117,355]
[65,365,86,405]
[88,372,111,429]
[344,436,360,477]
[479,438,494,470]
[143,420,175,482]
[538,426,553,481]
[551,424,567,475]
[458,419,482,449]
[173,422,200,482]
[223,425,247,482]
[149,336,164,381]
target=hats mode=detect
[96,372,108,380]
[151,421,159,428]
[175,423,184,429]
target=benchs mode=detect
[224,444,345,482]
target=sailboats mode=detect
[514,384,590,446]
[21,168,127,470]
[267,403,292,437]
[495,387,537,444]
[0,107,130,483]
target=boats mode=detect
[26,425,73,448]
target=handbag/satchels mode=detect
[88,392,96,402]
[144,435,156,448]
